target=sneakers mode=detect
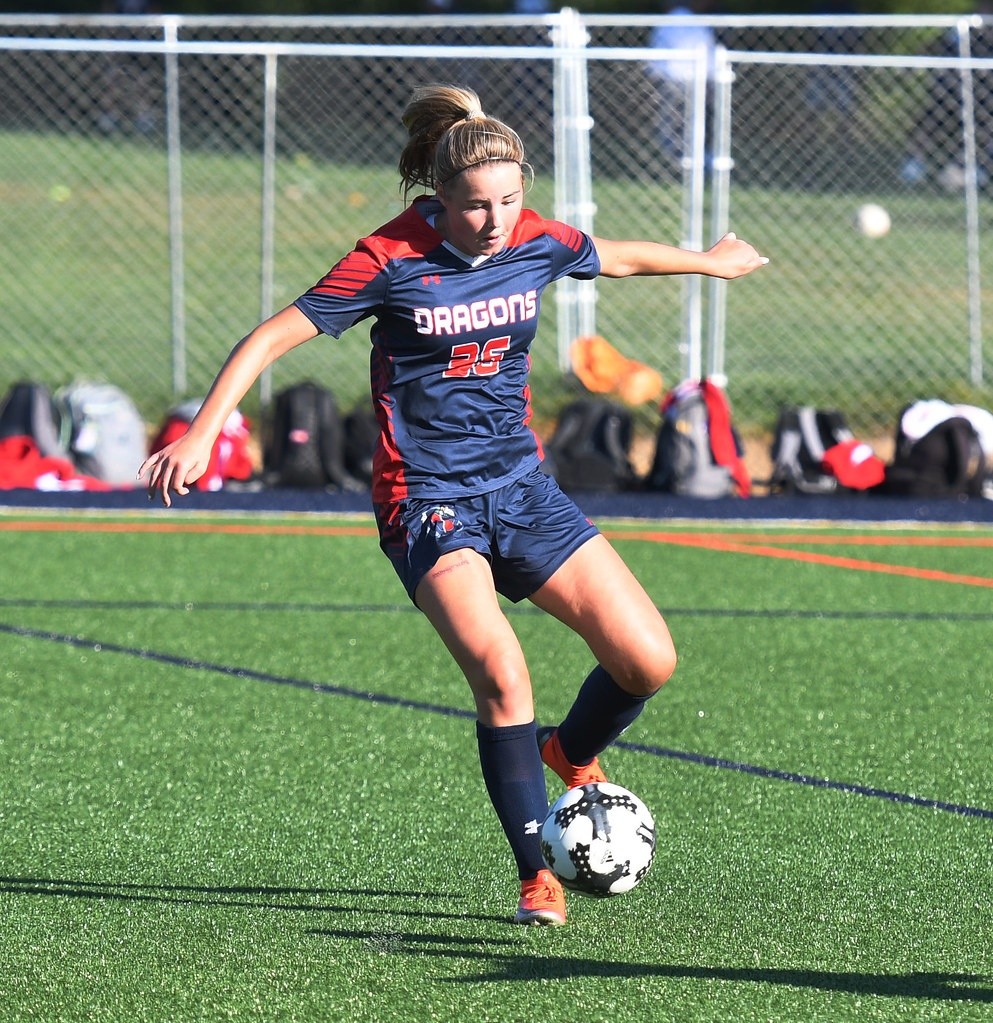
[516,873,565,925]
[536,727,607,792]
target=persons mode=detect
[135,79,772,924]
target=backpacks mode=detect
[0,379,987,502]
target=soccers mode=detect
[539,782,658,899]
